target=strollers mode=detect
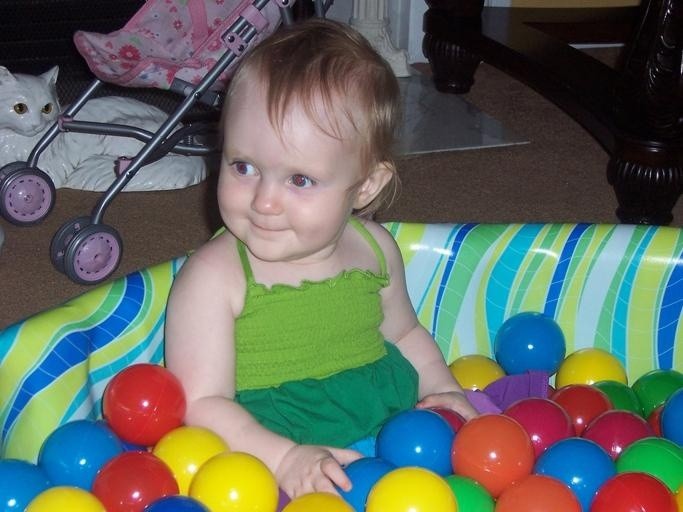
[0,0,343,284]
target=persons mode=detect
[162,17,473,509]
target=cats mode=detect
[0,64,209,193]
